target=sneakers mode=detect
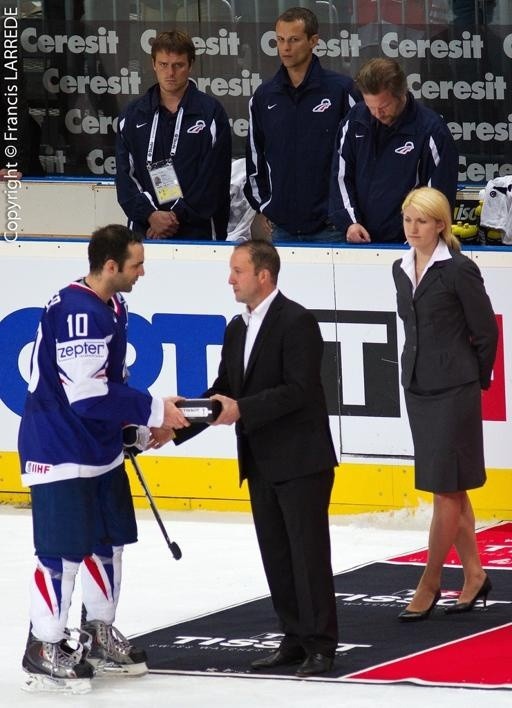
[73,622,149,665]
[24,635,96,676]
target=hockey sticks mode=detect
[129,451,182,562]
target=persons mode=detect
[396,189,502,623]
[242,6,364,243]
[327,57,458,249]
[115,30,232,245]
[140,238,340,678]
[16,222,190,694]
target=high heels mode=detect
[450,574,493,614]
[397,588,444,625]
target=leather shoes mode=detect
[297,654,334,679]
[250,652,294,669]
